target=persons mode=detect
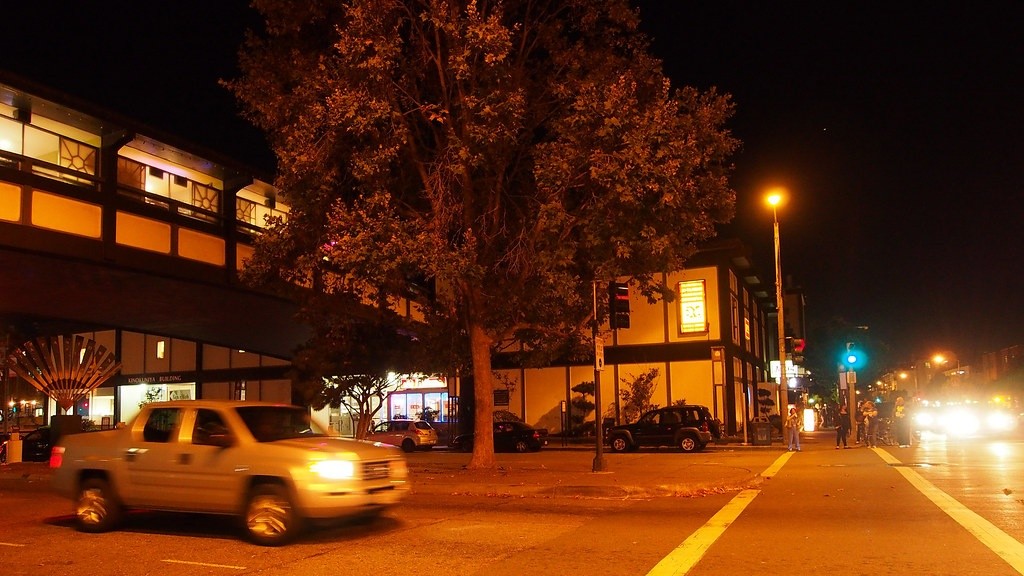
[785,408,803,452]
[812,397,912,450]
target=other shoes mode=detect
[789,447,800,451]
[836,441,878,449]
